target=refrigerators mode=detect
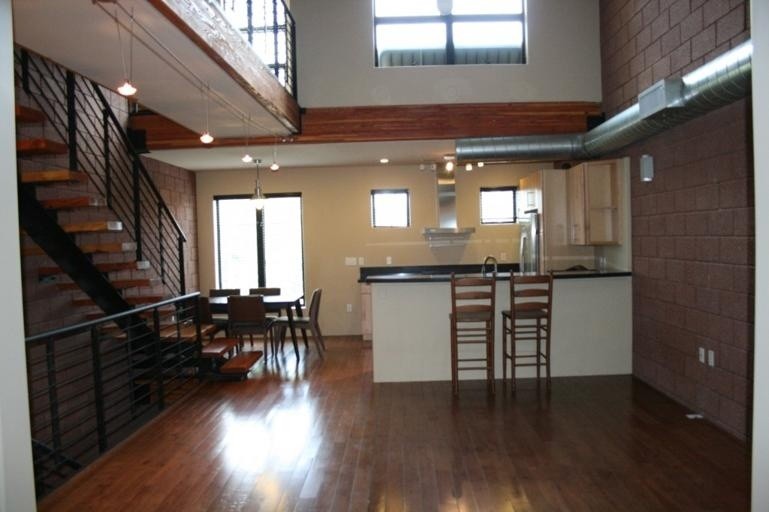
[516,213,539,274]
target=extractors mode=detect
[423,162,475,238]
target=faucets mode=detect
[482,256,498,276]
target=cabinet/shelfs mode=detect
[569,159,623,246]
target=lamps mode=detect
[639,154,655,182]
[253,158,264,211]
[106,0,284,171]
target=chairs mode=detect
[502,269,554,392]
[174,286,326,364]
[449,271,496,396]
